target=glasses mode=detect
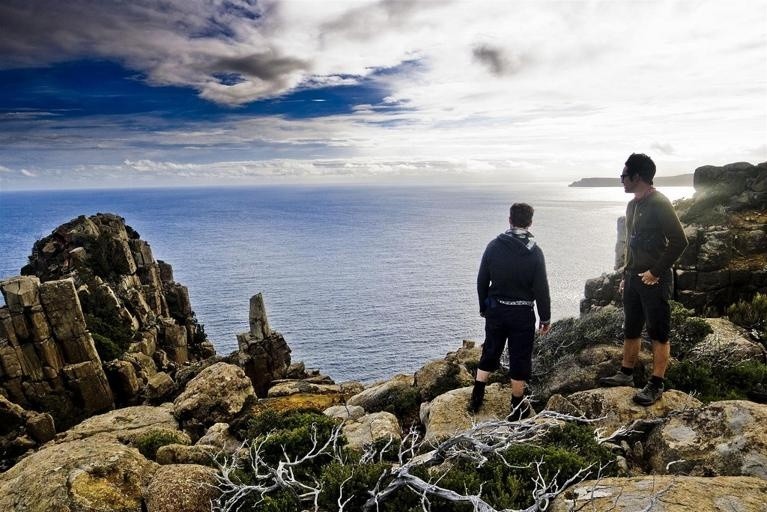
[619,173,629,183]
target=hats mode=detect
[624,151,655,186]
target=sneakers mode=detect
[507,404,530,422]
[466,392,483,413]
[598,371,635,387]
[632,380,665,406]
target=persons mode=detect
[465,202,552,424]
[595,152,689,408]
